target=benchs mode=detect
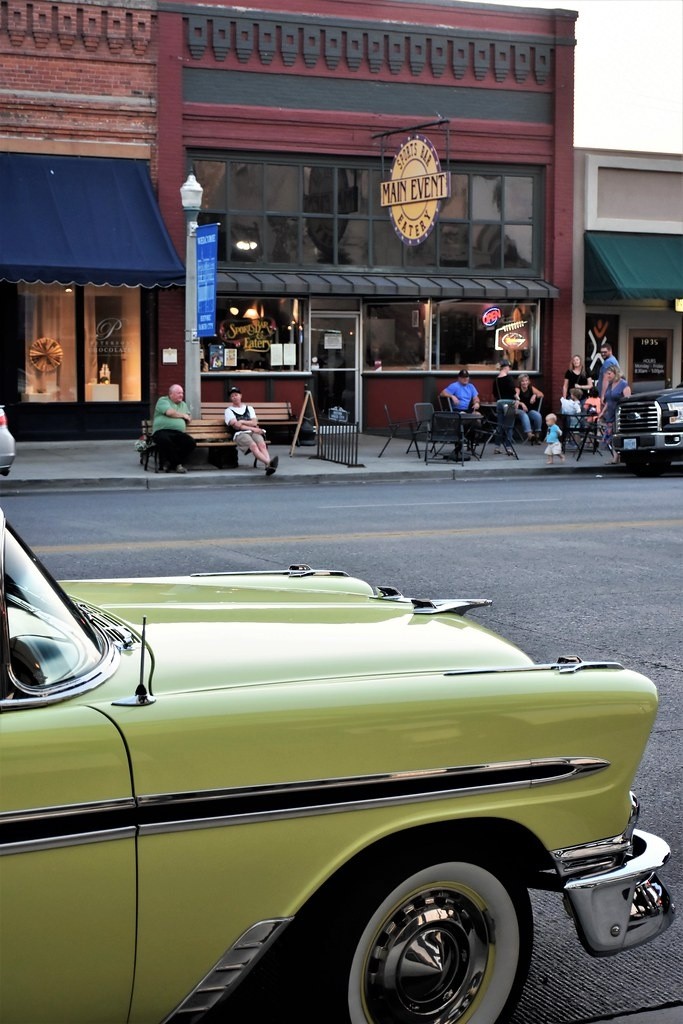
[139,421,273,472]
[201,402,300,448]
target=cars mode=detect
[0,500,678,1024]
[611,382,683,478]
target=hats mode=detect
[499,359,512,366]
[458,369,469,377]
[229,387,240,394]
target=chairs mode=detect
[439,395,480,448]
[513,396,546,446]
[378,405,436,460]
[474,403,519,460]
[576,419,615,461]
[407,403,454,455]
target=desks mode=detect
[562,413,597,451]
[434,414,484,459]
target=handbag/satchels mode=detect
[561,396,581,415]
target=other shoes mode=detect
[177,464,186,473]
[505,450,511,455]
[528,434,535,445]
[494,449,502,454]
[605,460,619,465]
[265,456,279,476]
[537,440,541,445]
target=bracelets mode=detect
[516,398,520,401]
[475,402,479,404]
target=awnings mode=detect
[585,227,683,303]
[0,150,186,288]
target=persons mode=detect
[562,343,631,464]
[515,374,547,440]
[151,383,198,475]
[544,414,566,464]
[223,385,279,477]
[493,363,520,457]
[440,369,480,463]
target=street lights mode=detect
[179,164,204,422]
